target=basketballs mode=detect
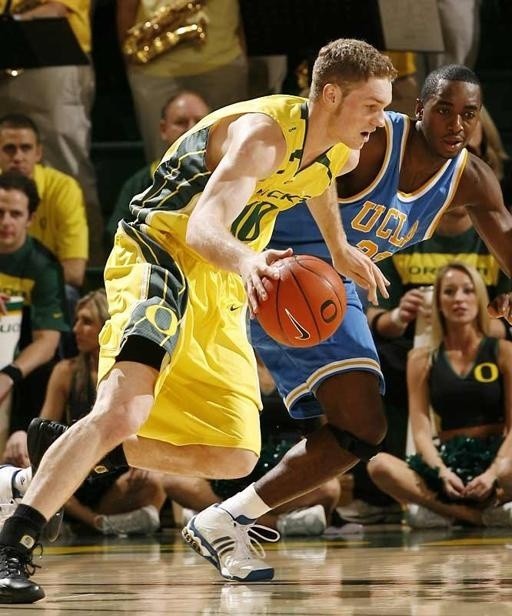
[255,256,347,348]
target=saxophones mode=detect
[122,0,209,65]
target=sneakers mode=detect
[102,505,160,536]
[481,501,512,527]
[181,503,273,580]
[0,464,22,530]
[0,543,45,604]
[409,503,454,528]
[277,504,326,535]
[27,418,71,541]
[335,498,403,523]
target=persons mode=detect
[182,65,512,585]
[1,38,396,601]
[0,1,511,538]
